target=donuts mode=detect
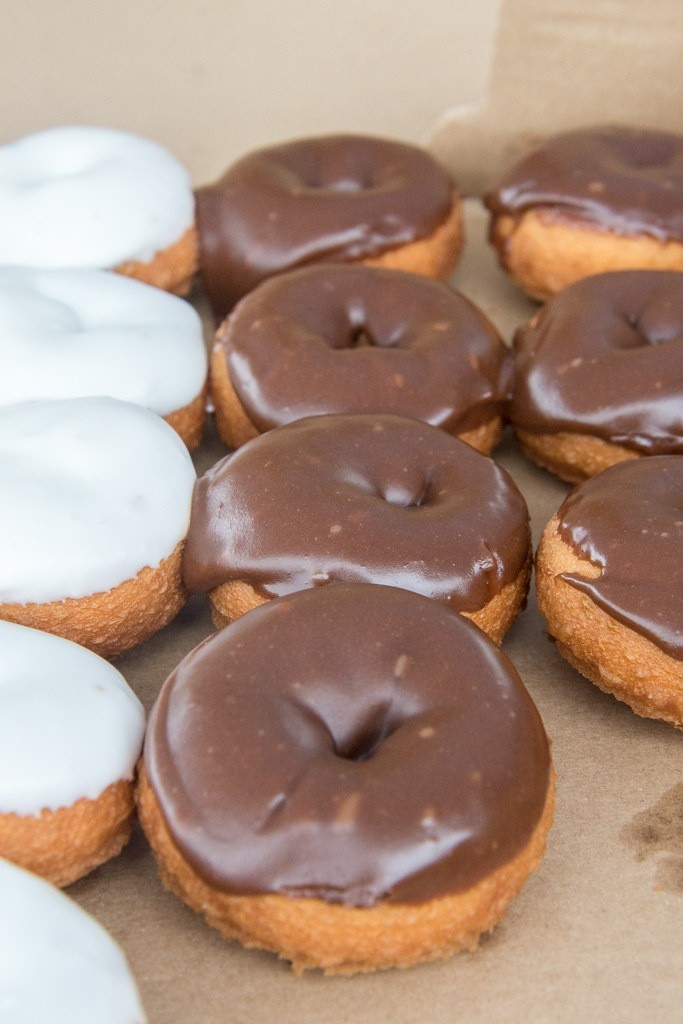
[0,123,683,1023]
